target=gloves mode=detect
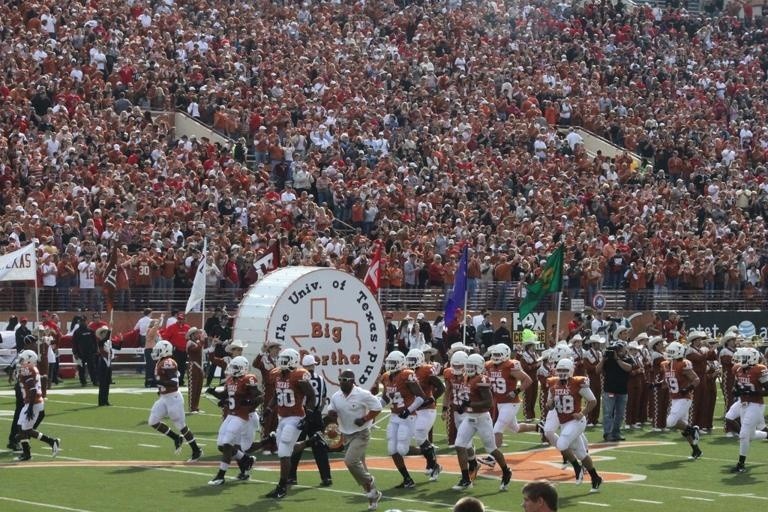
[677,386,694,397]
[145,376,161,387]
[421,395,435,407]
[259,406,272,427]
[297,408,332,431]
[354,417,367,426]
[648,381,662,394]
[205,386,220,398]
[399,408,411,419]
[731,388,756,397]
[457,398,471,415]
[24,409,36,421]
[507,388,521,400]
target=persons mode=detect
[1,305,767,512]
[0,2,768,311]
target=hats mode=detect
[420,343,439,356]
[301,355,320,366]
[536,349,558,362]
[18,305,232,331]
[523,335,540,346]
[95,325,109,340]
[445,341,475,355]
[556,325,749,351]
[185,327,203,341]
[23,334,39,346]
[260,338,283,353]
[224,339,249,354]
[382,311,508,323]
[1,180,767,267]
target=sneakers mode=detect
[394,477,415,489]
[261,431,280,456]
[52,438,61,458]
[365,474,375,499]
[476,454,496,467]
[320,478,332,487]
[368,490,383,511]
[562,454,590,485]
[687,450,704,460]
[286,476,299,484]
[266,485,286,499]
[589,476,604,493]
[499,468,513,492]
[237,455,257,482]
[424,443,443,482]
[186,449,205,464]
[311,429,329,449]
[730,461,746,473]
[451,462,481,491]
[189,409,206,415]
[414,416,764,449]
[6,439,33,461]
[208,476,225,486]
[174,434,184,455]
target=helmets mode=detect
[664,340,684,361]
[384,350,406,376]
[405,350,425,368]
[150,340,174,361]
[449,351,468,377]
[229,355,249,377]
[730,347,761,368]
[490,342,512,366]
[555,358,574,382]
[277,348,299,368]
[18,349,39,365]
[465,352,485,377]
